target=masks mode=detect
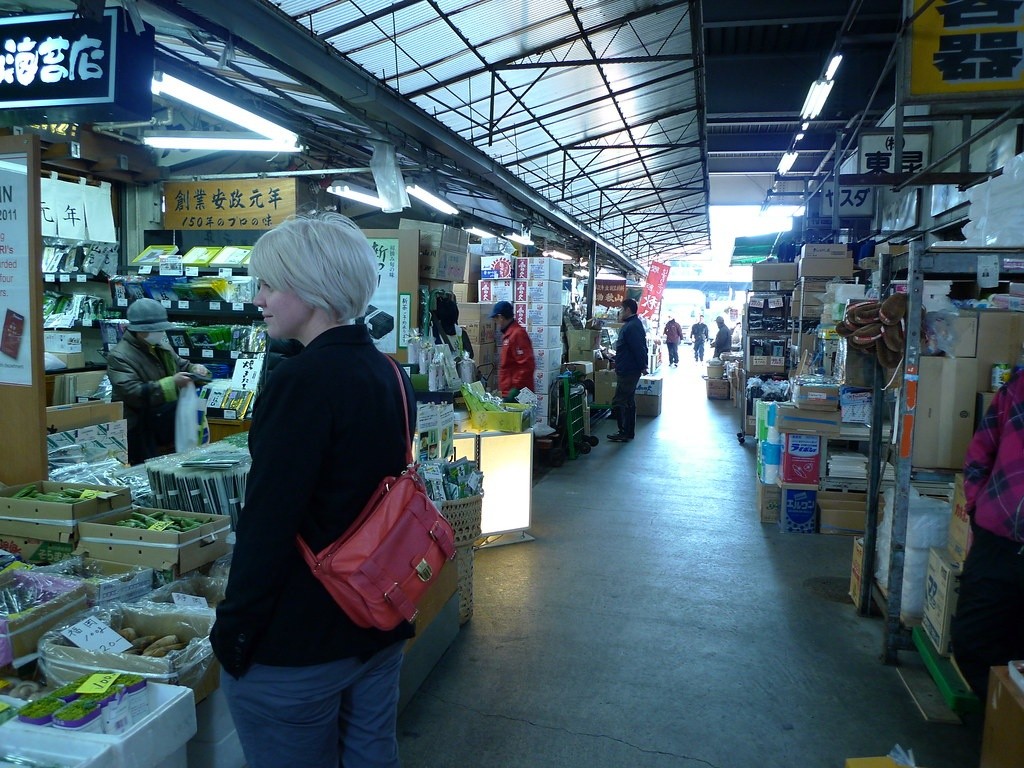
[140,332,164,345]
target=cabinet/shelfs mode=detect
[790,277,859,370]
[737,289,794,443]
[42,272,109,374]
[857,241,1024,665]
[106,265,263,420]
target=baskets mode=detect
[454,543,474,624]
[434,492,482,546]
[840,392,872,424]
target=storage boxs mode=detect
[44,331,85,368]
[399,218,663,426]
[0,480,246,768]
[706,244,1024,657]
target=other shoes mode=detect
[607,434,627,442]
[674,364,677,367]
[628,433,634,439]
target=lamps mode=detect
[143,70,310,152]
[327,180,381,208]
[405,182,460,215]
[775,53,842,176]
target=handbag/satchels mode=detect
[312,464,458,632]
[174,379,212,453]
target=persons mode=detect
[607,299,649,440]
[488,300,535,402]
[209,213,415,767]
[690,315,709,361]
[664,315,684,367]
[954,369,1024,699]
[107,298,208,465]
[259,331,306,390]
[462,328,474,359]
[710,316,731,358]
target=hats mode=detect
[669,316,675,321]
[125,298,177,332]
[488,301,514,317]
[714,316,724,323]
